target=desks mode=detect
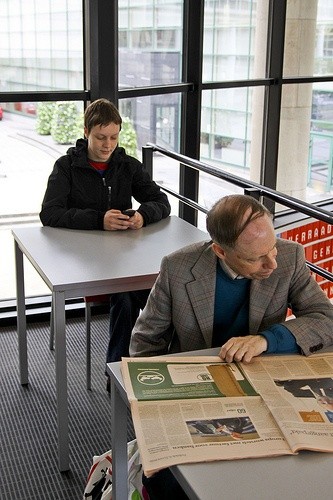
[12,215,211,473]
[106,346,333,500]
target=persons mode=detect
[127,194,333,500]
[40,98,171,395]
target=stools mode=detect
[48,294,112,391]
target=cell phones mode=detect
[117,210,136,221]
